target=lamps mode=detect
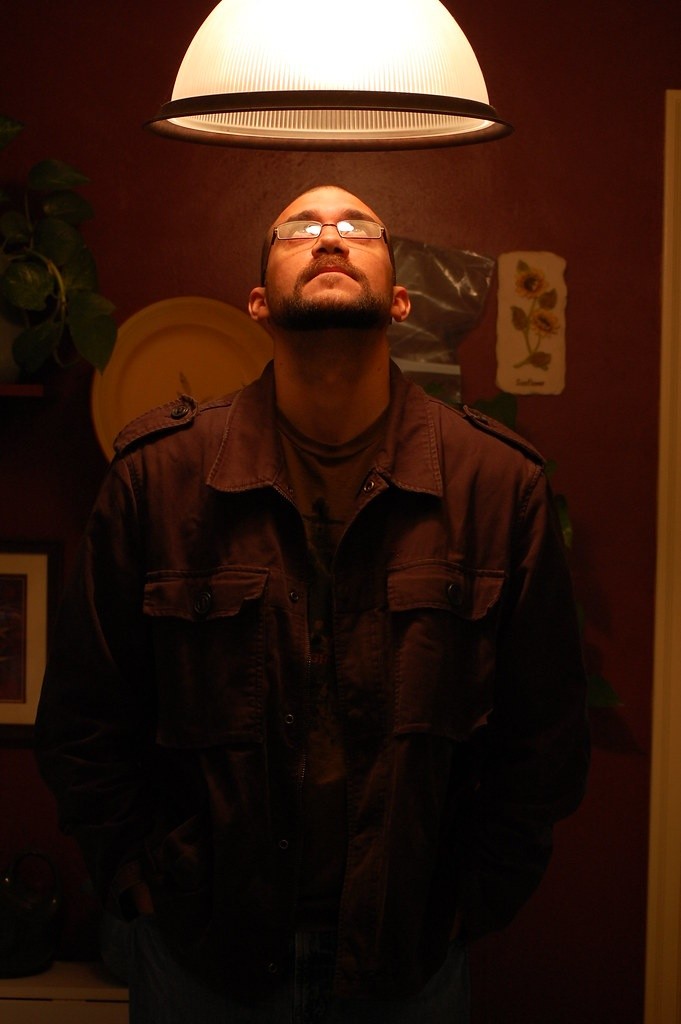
[141,0,514,152]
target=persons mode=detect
[34,183,594,1024]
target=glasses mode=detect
[261,219,396,286]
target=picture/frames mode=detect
[0,540,64,747]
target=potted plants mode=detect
[0,112,116,384]
